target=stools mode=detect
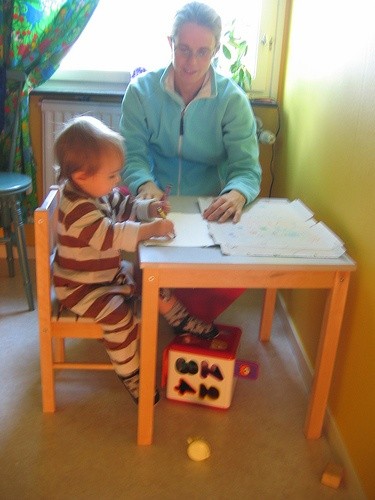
[0,170,36,311]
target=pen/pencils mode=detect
[157,207,175,237]
[157,184,170,212]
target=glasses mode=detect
[176,42,215,59]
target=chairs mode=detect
[35,184,118,413]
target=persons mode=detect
[53,113,222,407]
[119,2,262,223]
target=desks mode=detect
[138,196,357,446]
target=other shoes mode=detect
[118,368,161,408]
[173,315,220,341]
